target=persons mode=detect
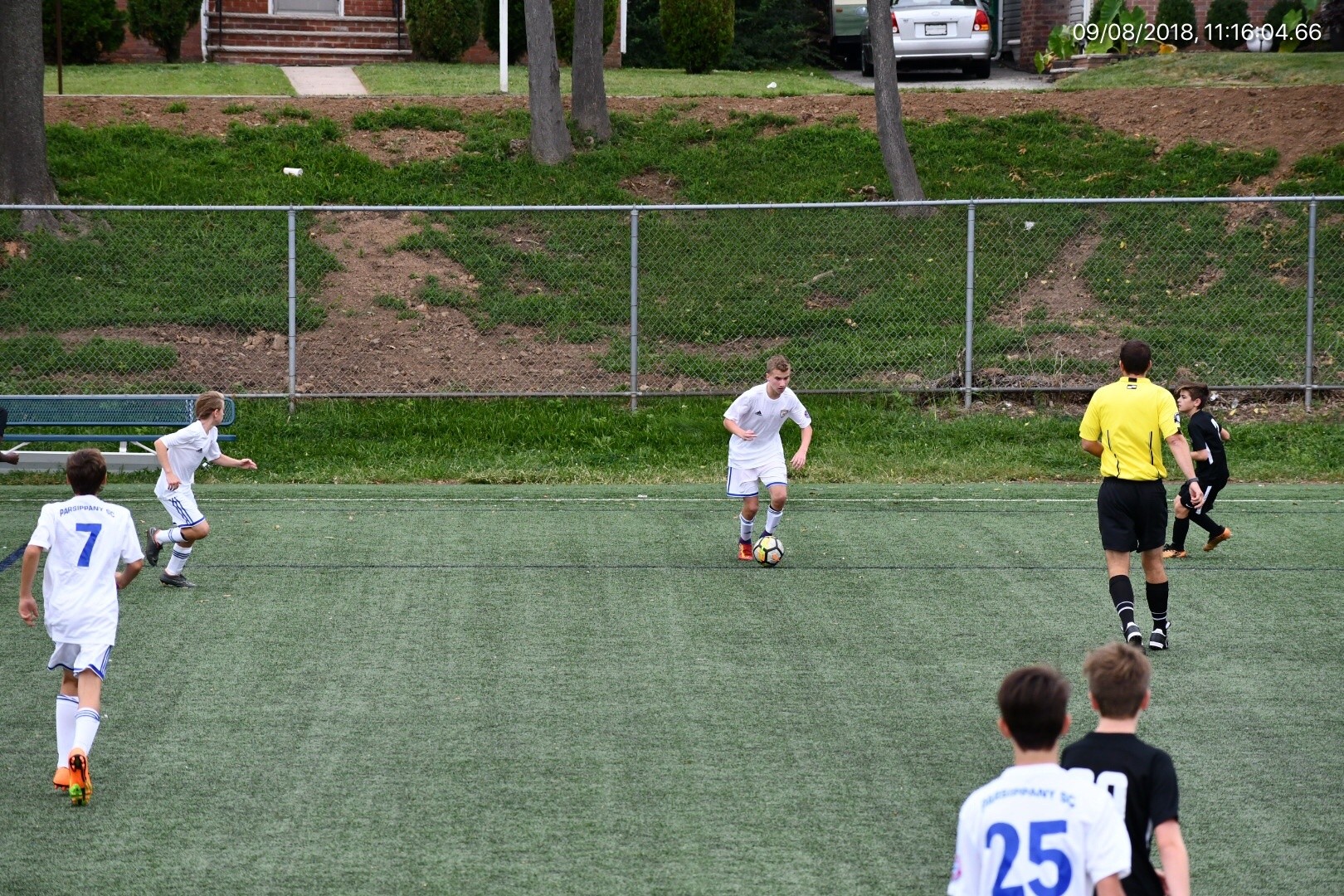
[723,355,814,560]
[1163,382,1232,558]
[19,448,145,807]
[145,391,258,587]
[1059,641,1191,896]
[0,408,19,465]
[1079,340,1204,649]
[947,667,1132,896]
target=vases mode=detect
[1007,39,1022,61]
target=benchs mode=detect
[0,394,238,473]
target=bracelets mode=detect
[1187,477,1199,484]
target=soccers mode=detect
[753,535,784,568]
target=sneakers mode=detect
[1162,544,1186,558]
[1149,622,1171,650]
[160,570,196,587]
[146,527,163,565]
[1203,527,1231,551]
[1120,622,1146,655]
[53,767,69,792]
[68,747,92,806]
[739,537,753,560]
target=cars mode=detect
[859,0,994,78]
